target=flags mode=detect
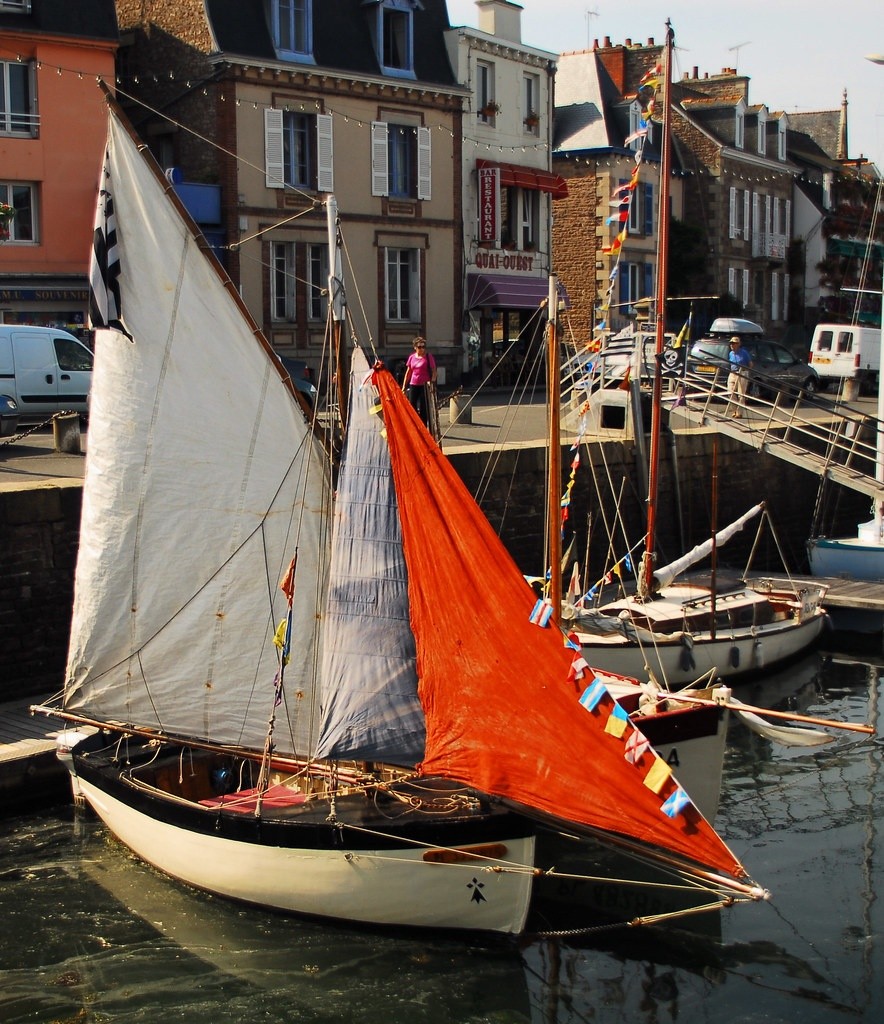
[528,40,693,819]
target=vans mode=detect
[808,323,881,394]
[0,322,95,437]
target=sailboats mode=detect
[802,157,883,588]
[436,27,831,689]
[26,73,775,949]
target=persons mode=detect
[726,337,753,418]
[401,336,438,429]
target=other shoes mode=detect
[732,413,742,418]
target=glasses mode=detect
[416,345,426,349]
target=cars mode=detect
[276,352,311,383]
[290,376,319,418]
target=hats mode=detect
[729,337,740,343]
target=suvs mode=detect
[684,317,821,404]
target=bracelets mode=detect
[431,381,434,384]
